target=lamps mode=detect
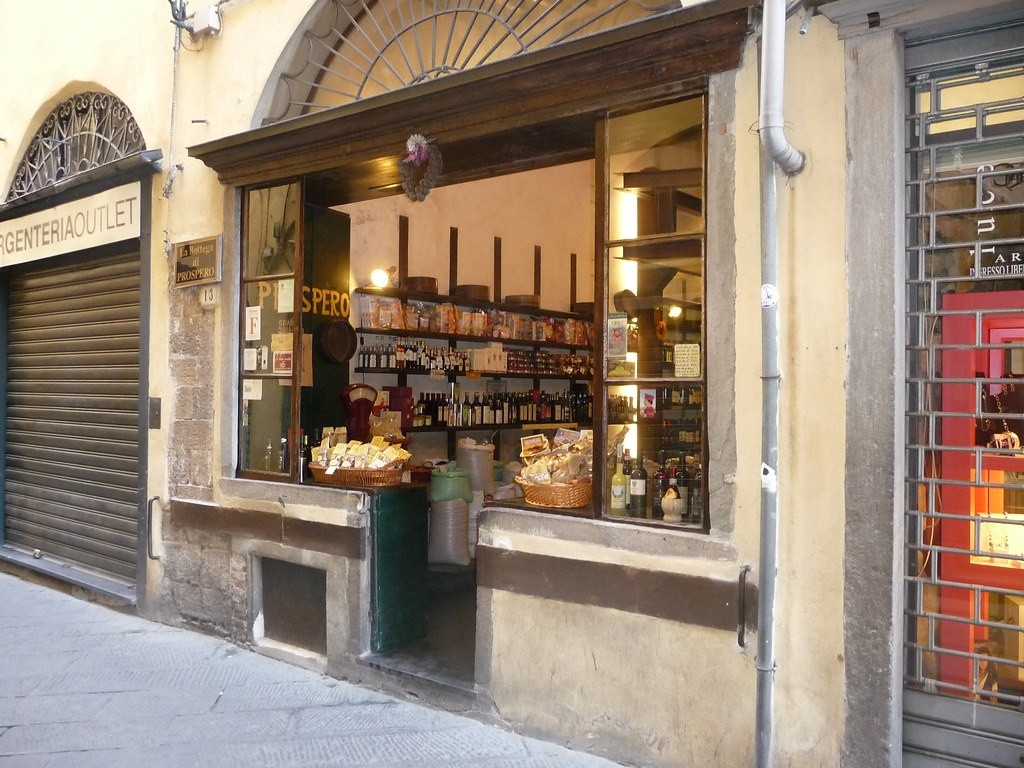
[371,266,397,288]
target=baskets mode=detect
[308,459,410,487]
[514,475,593,509]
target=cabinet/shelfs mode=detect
[356,286,595,433]
[662,328,702,450]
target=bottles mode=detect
[630,454,647,518]
[662,339,701,369]
[695,464,702,497]
[358,336,470,371]
[265,439,274,471]
[300,429,311,483]
[653,452,668,520]
[539,389,593,423]
[279,433,289,472]
[611,444,627,516]
[462,392,471,426]
[313,429,321,447]
[482,392,537,424]
[660,417,702,479]
[665,467,681,499]
[608,394,634,423]
[623,449,631,509]
[471,392,482,425]
[418,393,452,427]
[675,453,690,518]
